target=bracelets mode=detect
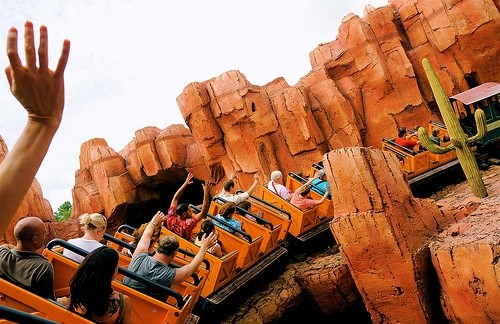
[151,220,157,226]
[412,133,414,135]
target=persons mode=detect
[121,221,161,258]
[289,172,328,212]
[165,173,212,244]
[396,127,421,160]
[121,211,218,303]
[211,202,241,234]
[213,171,259,206]
[68,246,131,324]
[268,171,294,202]
[0,21,71,238]
[194,220,225,259]
[63,213,107,264]
[430,130,450,146]
[236,201,263,223]
[0,217,57,303]
[309,169,331,196]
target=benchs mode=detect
[0,121,458,324]
